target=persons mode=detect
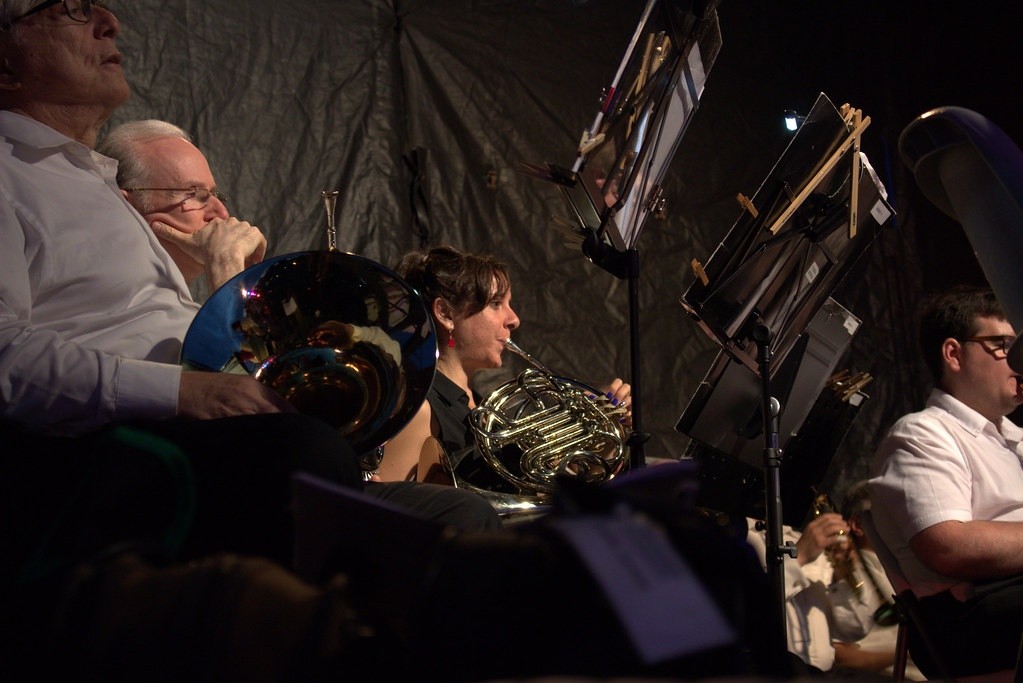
[91,116,507,536]
[0,0,369,494]
[38,480,798,683]
[867,288,1023,683]
[740,474,928,683]
[366,244,679,496]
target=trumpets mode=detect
[809,481,901,626]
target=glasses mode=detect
[954,335,1017,359]
[120,187,228,204]
[0,0,97,33]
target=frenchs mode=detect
[416,250,634,527]
[174,187,439,487]
